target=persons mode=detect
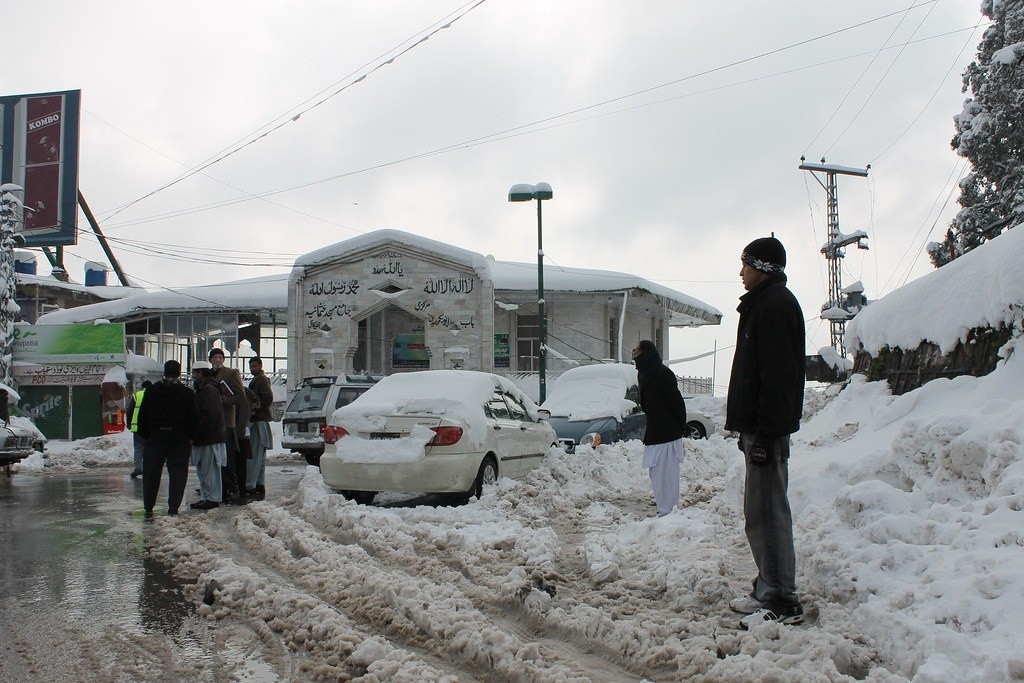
[724,232,806,631]
[126,348,274,521]
[631,339,686,520]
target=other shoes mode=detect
[190,500,219,509]
[168,509,178,516]
[143,512,154,521]
[130,471,143,477]
[224,485,265,506]
[195,489,200,492]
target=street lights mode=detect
[507,182,553,404]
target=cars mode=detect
[539,362,716,455]
[320,370,560,505]
[0,388,38,467]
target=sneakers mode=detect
[740,608,805,633]
[729,593,769,614]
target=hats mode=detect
[141,380,152,388]
[741,231,787,274]
[192,361,212,369]
[164,360,181,378]
[209,348,224,362]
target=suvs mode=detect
[281,373,387,467]
